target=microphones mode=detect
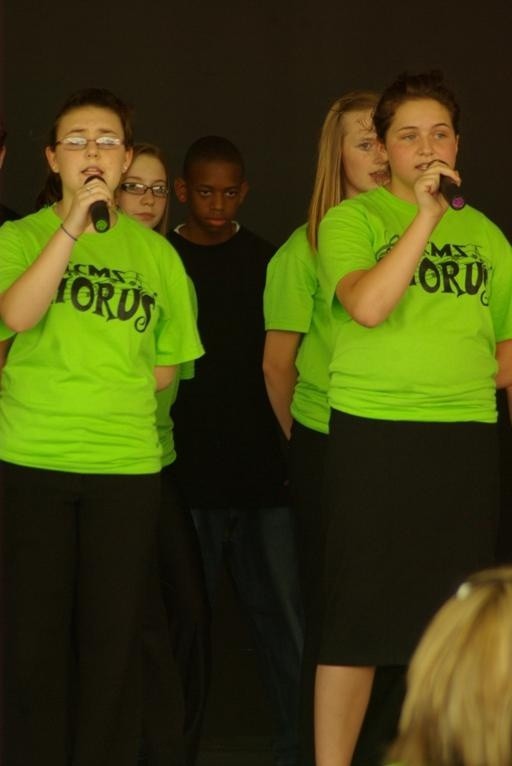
[84,176,110,232]
[428,160,464,210]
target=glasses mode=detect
[52,135,127,150]
[117,182,171,198]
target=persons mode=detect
[1,106,23,228]
[0,83,195,765]
[301,69,512,766]
[110,133,214,766]
[375,563,512,766]
[264,85,419,765]
[161,132,303,763]
